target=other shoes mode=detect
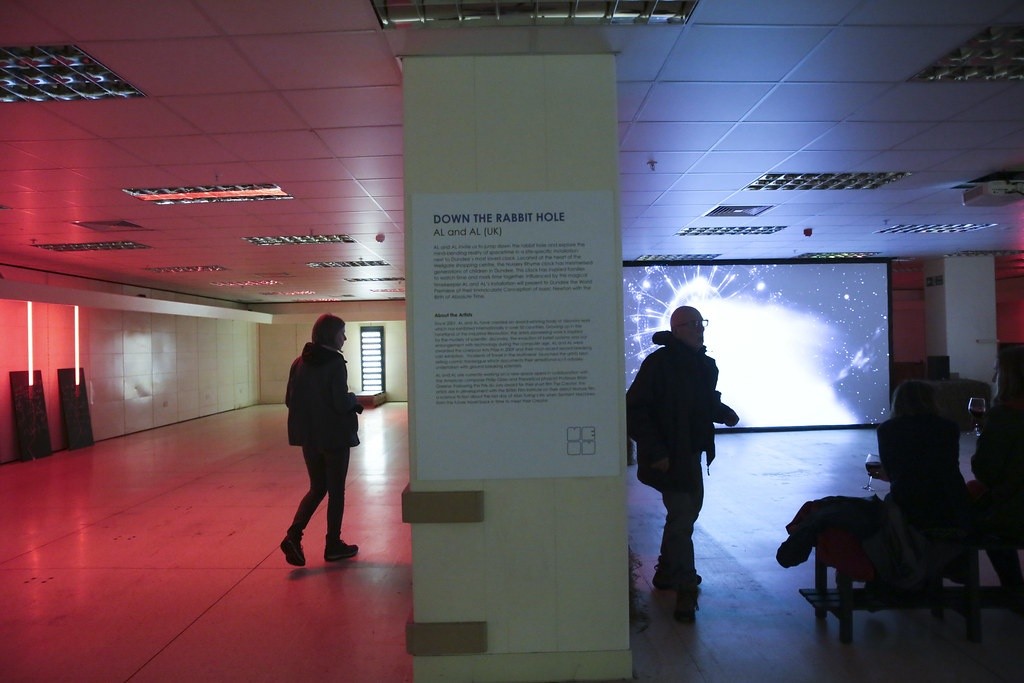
[651,564,702,591]
[672,584,701,623]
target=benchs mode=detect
[786,501,1024,644]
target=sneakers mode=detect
[280,533,306,566]
[324,532,358,561]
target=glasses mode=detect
[672,319,708,329]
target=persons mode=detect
[878,381,989,642]
[281,314,365,567]
[626,305,740,623]
[970,346,1024,613]
